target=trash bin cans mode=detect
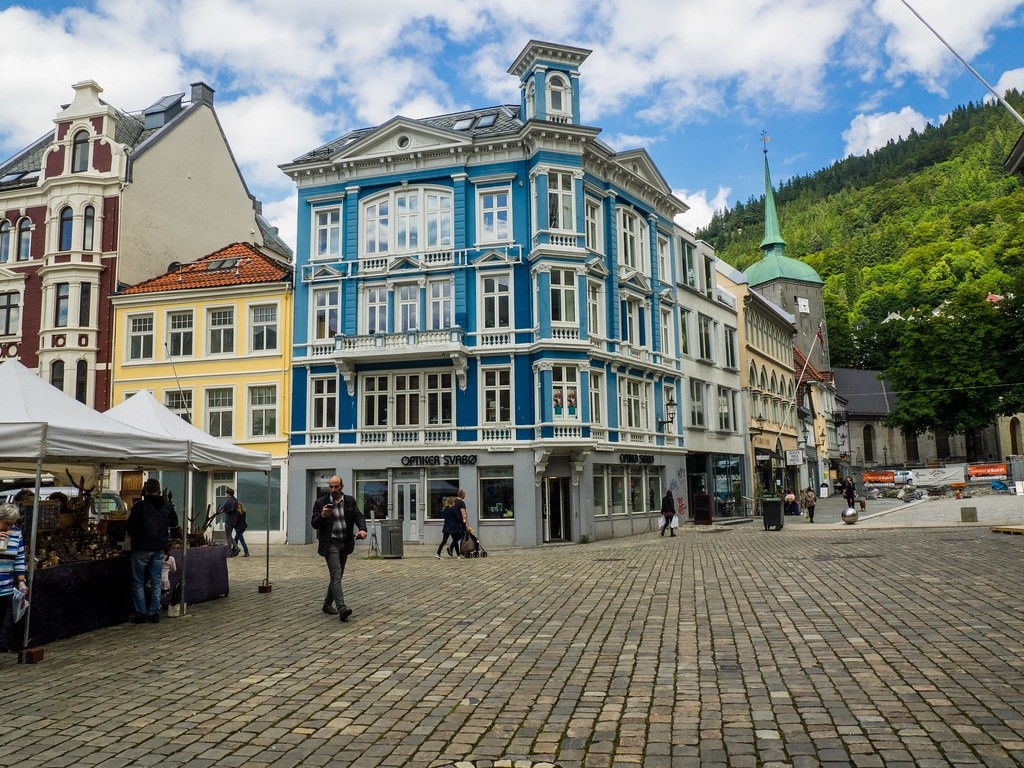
[379,519,404,558]
[762,498,784,531]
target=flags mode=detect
[816,322,825,356]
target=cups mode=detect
[0,533,10,552]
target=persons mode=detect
[233,504,250,557]
[435,490,470,559]
[842,476,857,509]
[0,503,28,656]
[804,487,817,523]
[310,475,367,621]
[217,489,241,558]
[125,479,179,625]
[659,490,677,537]
[145,545,177,612]
[785,489,801,515]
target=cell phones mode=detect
[327,504,333,509]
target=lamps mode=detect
[657,394,677,431]
[749,413,765,441]
[838,433,848,446]
[815,431,826,448]
[798,426,809,447]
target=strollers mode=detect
[465,530,489,557]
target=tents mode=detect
[0,359,272,665]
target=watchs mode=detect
[18,578,26,583]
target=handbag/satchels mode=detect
[462,532,474,552]
[12,587,29,624]
[671,515,679,529]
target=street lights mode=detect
[883,445,888,467]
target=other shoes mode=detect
[131,613,149,624]
[226,550,231,557]
[323,604,338,614]
[148,614,159,622]
[233,549,241,556]
[435,554,444,560]
[447,547,453,556]
[340,609,352,620]
[242,553,249,557]
[457,554,465,559]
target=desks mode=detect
[10,543,229,653]
